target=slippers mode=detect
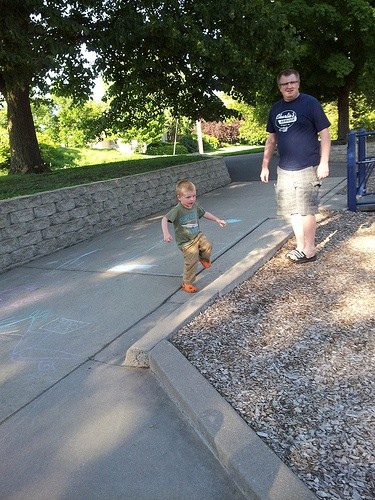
[181,282,197,293]
[285,249,317,263]
[199,258,211,269]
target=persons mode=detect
[259,69,333,264]
[162,180,229,292]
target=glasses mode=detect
[279,80,299,86]
